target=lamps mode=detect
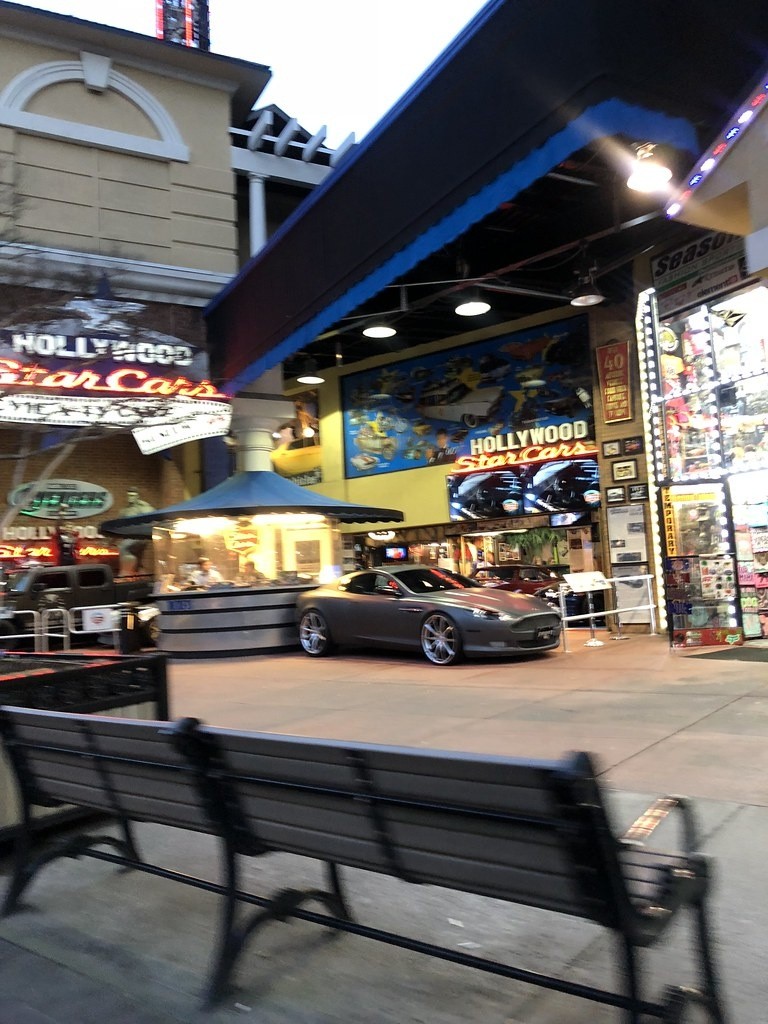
[362,319,397,338]
[297,358,326,385]
[627,154,673,192]
[455,294,491,317]
[570,273,605,307]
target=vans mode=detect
[6,563,114,619]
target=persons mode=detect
[238,561,265,583]
[473,549,486,571]
[189,556,225,587]
[108,485,156,576]
[56,501,79,565]
[489,552,496,579]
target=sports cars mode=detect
[294,563,564,665]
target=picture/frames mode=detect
[612,563,651,625]
[606,486,624,503]
[606,504,648,563]
[338,313,596,483]
[612,459,637,481]
[627,483,648,501]
[622,436,643,455]
[602,439,621,458]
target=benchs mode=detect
[0,705,735,1023]
